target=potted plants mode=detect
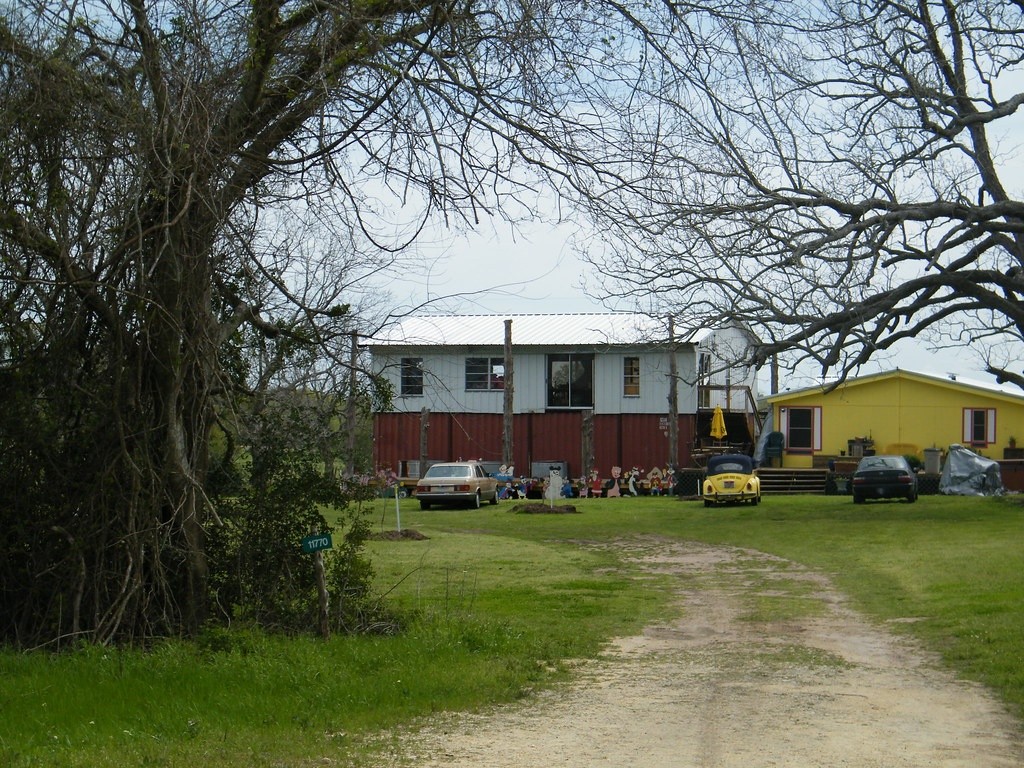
[863,446,877,456]
[828,457,836,471]
[1008,435,1016,448]
[834,478,849,491]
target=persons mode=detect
[467,370,504,389]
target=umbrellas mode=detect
[710,404,728,447]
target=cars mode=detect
[701,454,761,507]
[416,462,500,511]
[852,453,923,504]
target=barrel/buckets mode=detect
[923,448,941,473]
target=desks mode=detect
[702,446,733,450]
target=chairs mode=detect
[687,440,752,467]
[765,432,784,467]
